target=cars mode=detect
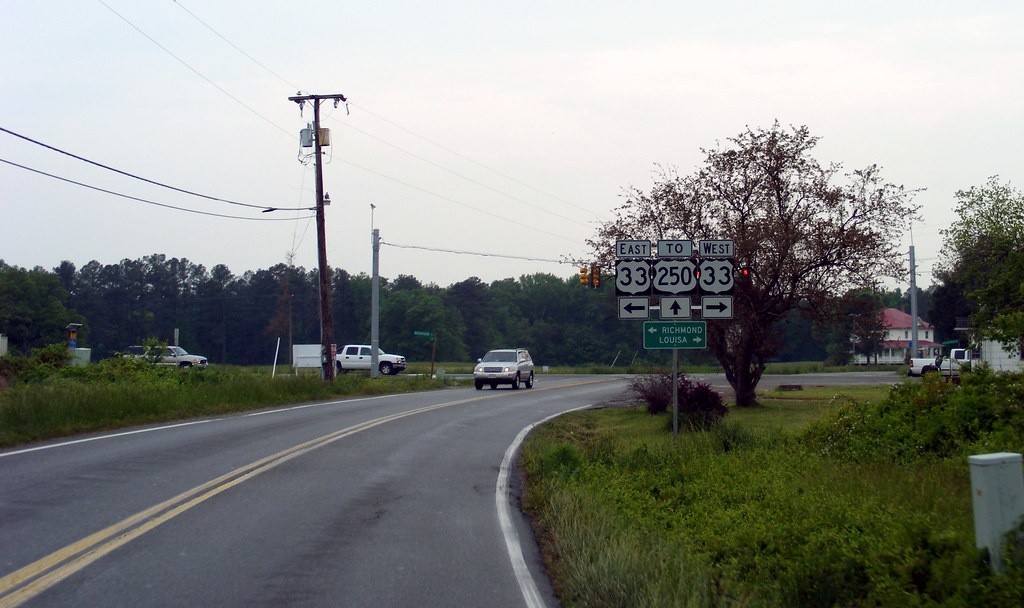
[121,346,150,364]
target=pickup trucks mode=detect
[907,347,980,383]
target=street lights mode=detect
[262,203,334,383]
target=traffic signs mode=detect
[614,238,652,321]
[697,238,736,321]
[642,320,708,350]
[652,239,700,321]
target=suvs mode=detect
[334,343,408,376]
[138,346,208,371]
[474,347,534,391]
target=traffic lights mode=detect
[589,266,601,288]
[580,267,589,285]
